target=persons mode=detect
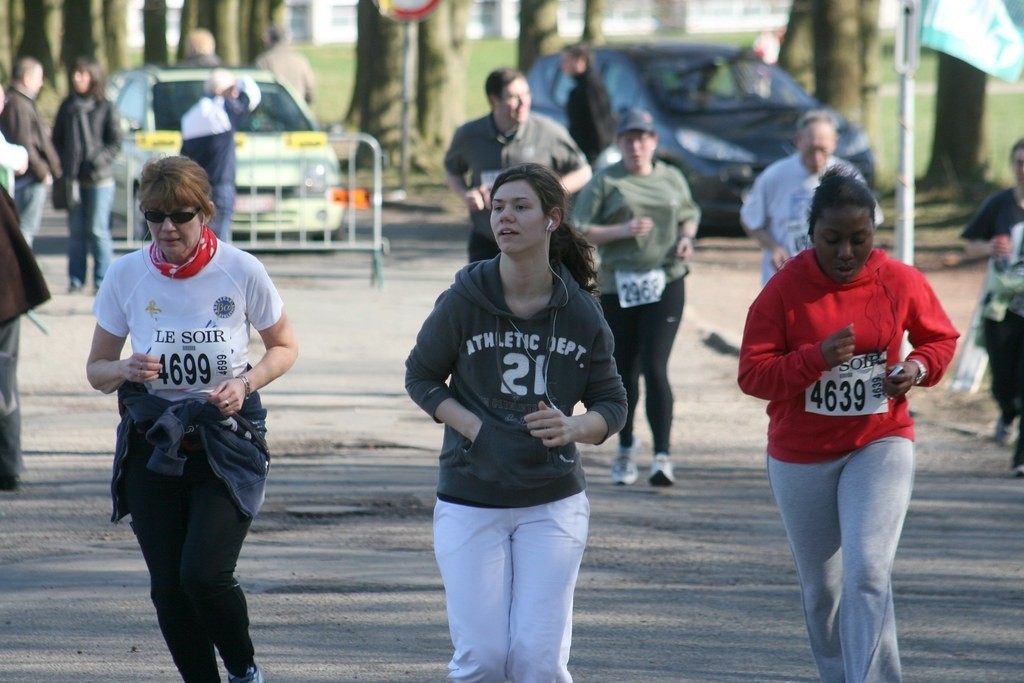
[51,56,122,296]
[0,83,29,200]
[180,71,247,239]
[0,185,52,490]
[562,43,615,162]
[739,108,884,293]
[444,67,592,262]
[0,57,62,245]
[86,157,299,683]
[943,139,1024,476]
[738,163,960,683]
[569,108,702,487]
[181,22,318,105]
[405,162,629,683]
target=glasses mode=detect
[144,207,200,224]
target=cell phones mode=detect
[888,365,905,383]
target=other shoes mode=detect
[996,415,1020,446]
[1011,457,1024,475]
[68,286,82,296]
[228,665,264,683]
[0,475,19,490]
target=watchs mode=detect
[908,359,927,385]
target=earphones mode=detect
[546,219,554,231]
[201,212,206,226]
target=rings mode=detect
[226,400,228,407]
[138,362,142,369]
[138,371,141,376]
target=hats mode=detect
[616,109,654,135]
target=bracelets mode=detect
[680,235,693,240]
[237,374,251,400]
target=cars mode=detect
[105,63,348,258]
[525,36,877,237]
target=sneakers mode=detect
[613,436,639,485]
[648,456,674,485]
